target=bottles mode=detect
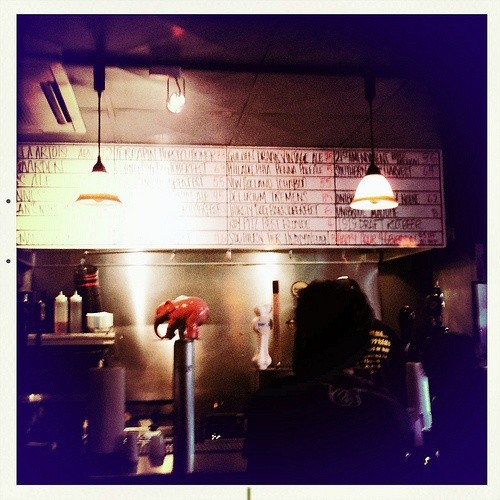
[54,291,68,334]
[70,290,82,333]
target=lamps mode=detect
[348,43,400,211]
[70,54,124,209]
[165,72,188,113]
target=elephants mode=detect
[154,296,212,341]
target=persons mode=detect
[244,278,414,485]
[399,302,487,454]
